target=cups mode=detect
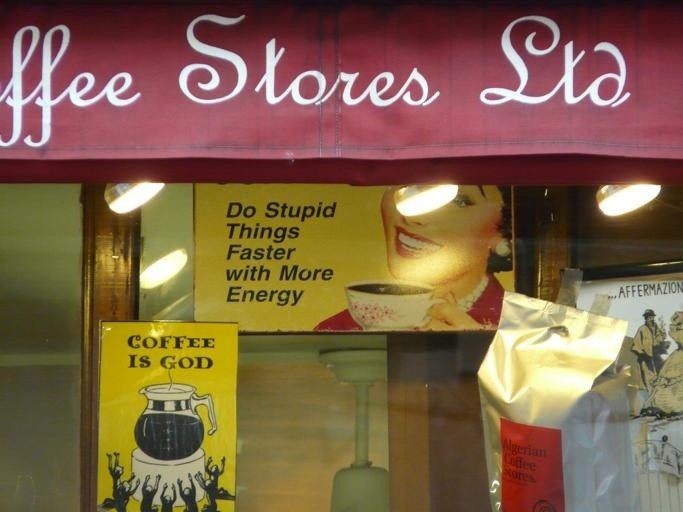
[346,282,446,331]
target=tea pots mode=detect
[134,384,217,459]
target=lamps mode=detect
[104,182,165,214]
[596,179,660,221]
[138,237,188,292]
[393,183,458,218]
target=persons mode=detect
[310,184,512,334]
[627,306,671,386]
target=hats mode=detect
[641,308,656,317]
